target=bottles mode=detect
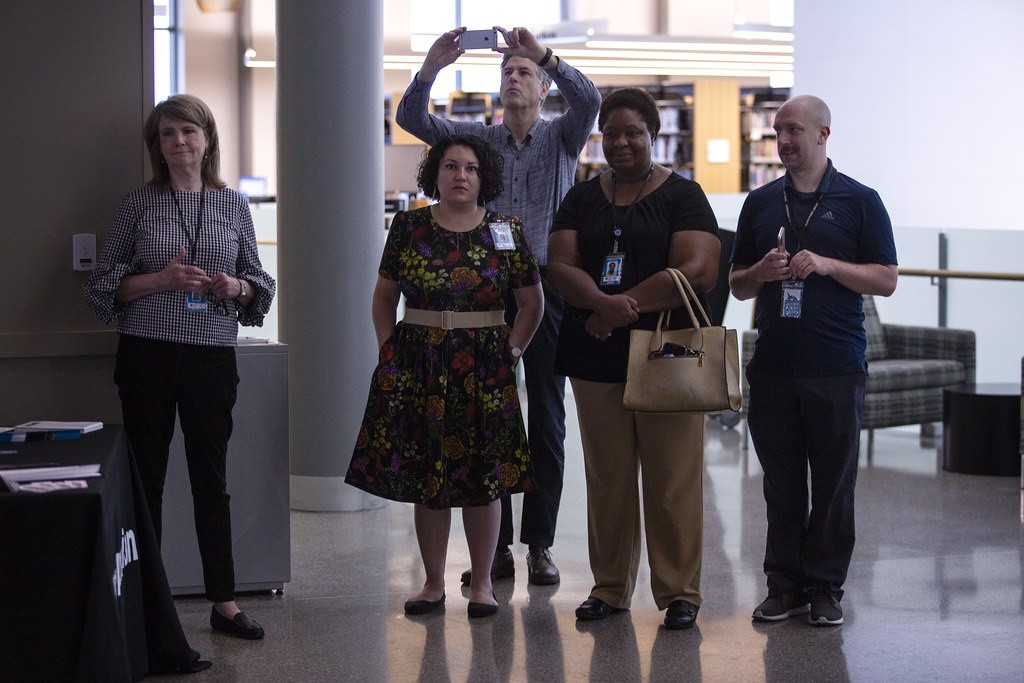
[397,190,409,212]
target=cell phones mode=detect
[459,30,498,50]
[777,226,786,259]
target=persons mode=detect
[79,94,277,638]
[607,263,616,277]
[546,86,719,629]
[727,95,897,628]
[396,26,603,585]
[344,134,545,619]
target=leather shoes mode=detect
[663,597,701,629]
[574,595,628,620]
[526,546,560,586]
[460,549,516,583]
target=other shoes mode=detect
[207,604,264,640]
[468,589,500,617]
[403,588,446,615]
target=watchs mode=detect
[538,46,553,67]
[236,276,248,304]
[508,347,523,359]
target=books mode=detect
[451,114,504,126]
[0,421,103,442]
[747,110,787,190]
[540,107,693,180]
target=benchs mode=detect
[737,293,977,459]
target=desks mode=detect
[0,422,213,683]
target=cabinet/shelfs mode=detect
[446,77,793,195]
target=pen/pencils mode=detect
[1,450,23,453]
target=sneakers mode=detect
[806,584,843,626]
[750,588,810,620]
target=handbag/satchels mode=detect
[620,268,742,413]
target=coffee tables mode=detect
[942,382,1024,477]
